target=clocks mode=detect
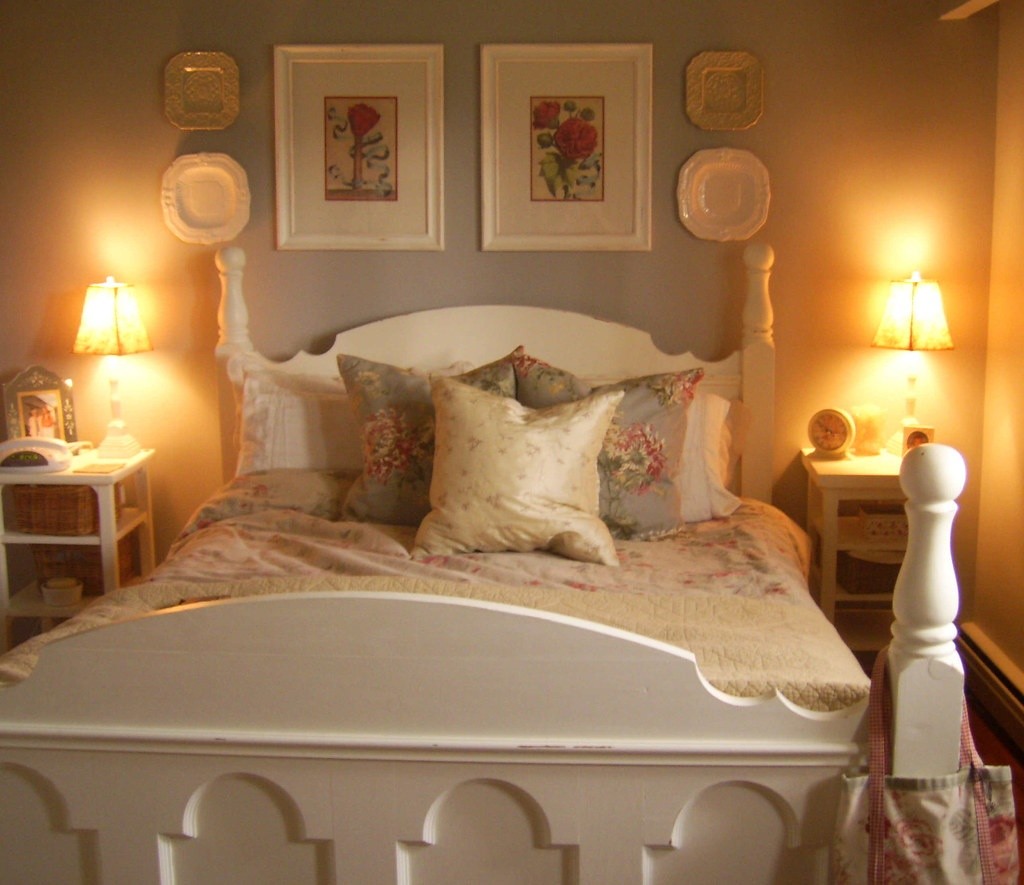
[809,409,857,457]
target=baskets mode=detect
[5,485,121,535]
[851,500,908,543]
[32,532,136,595]
[837,558,902,594]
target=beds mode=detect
[0,241,974,885]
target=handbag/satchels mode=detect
[833,648,1018,885]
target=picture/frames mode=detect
[0,364,78,467]
[273,44,446,252]
[478,42,654,252]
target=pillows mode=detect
[588,371,744,521]
[407,369,628,568]
[335,345,526,527]
[225,352,478,477]
[512,351,704,540]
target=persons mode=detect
[26,406,55,439]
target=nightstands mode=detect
[803,447,906,626]
[0,448,157,653]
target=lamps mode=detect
[73,277,155,462]
[874,271,960,461]
